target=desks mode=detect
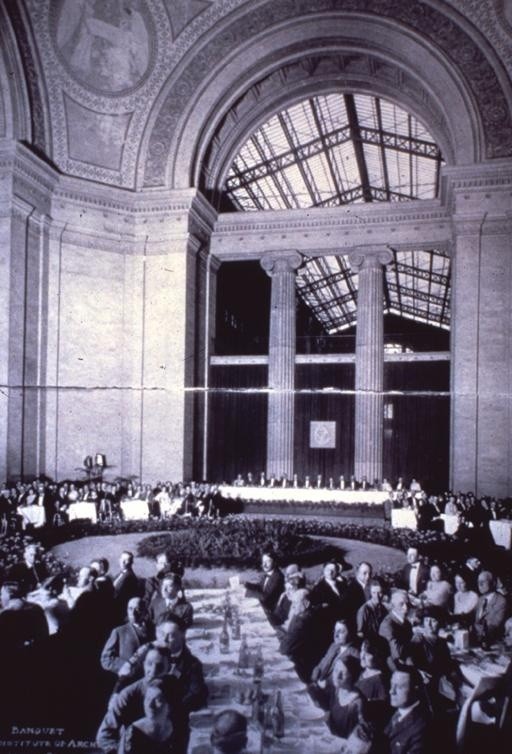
[16,485,512,754]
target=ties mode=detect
[392,711,401,729]
[482,598,487,613]
[166,603,172,610]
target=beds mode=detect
[16,485,512,754]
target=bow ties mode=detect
[411,566,416,568]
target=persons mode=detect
[0,467,512,754]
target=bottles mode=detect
[219,592,284,740]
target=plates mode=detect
[188,712,218,733]
[189,638,212,648]
[237,597,325,722]
